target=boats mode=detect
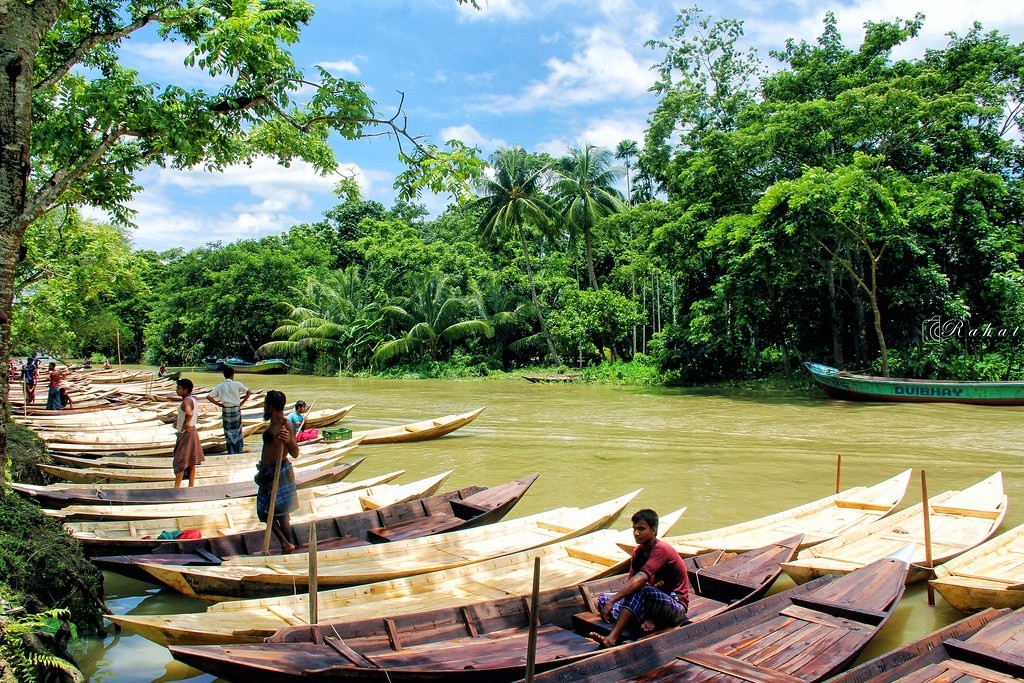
[521,374,580,384]
[820,604,1024,683]
[7,361,542,590]
[928,522,1024,615]
[222,355,292,375]
[133,488,646,607]
[614,467,915,597]
[510,543,917,683]
[318,406,488,446]
[166,532,807,683]
[802,361,1024,407]
[101,505,690,653]
[778,471,1009,586]
[202,354,224,372]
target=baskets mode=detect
[322,427,352,441]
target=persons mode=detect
[20,352,41,403]
[288,400,318,442]
[158,365,166,377]
[46,362,62,410]
[206,367,251,454]
[59,388,73,410]
[173,379,205,488]
[590,510,690,646]
[254,390,300,555]
